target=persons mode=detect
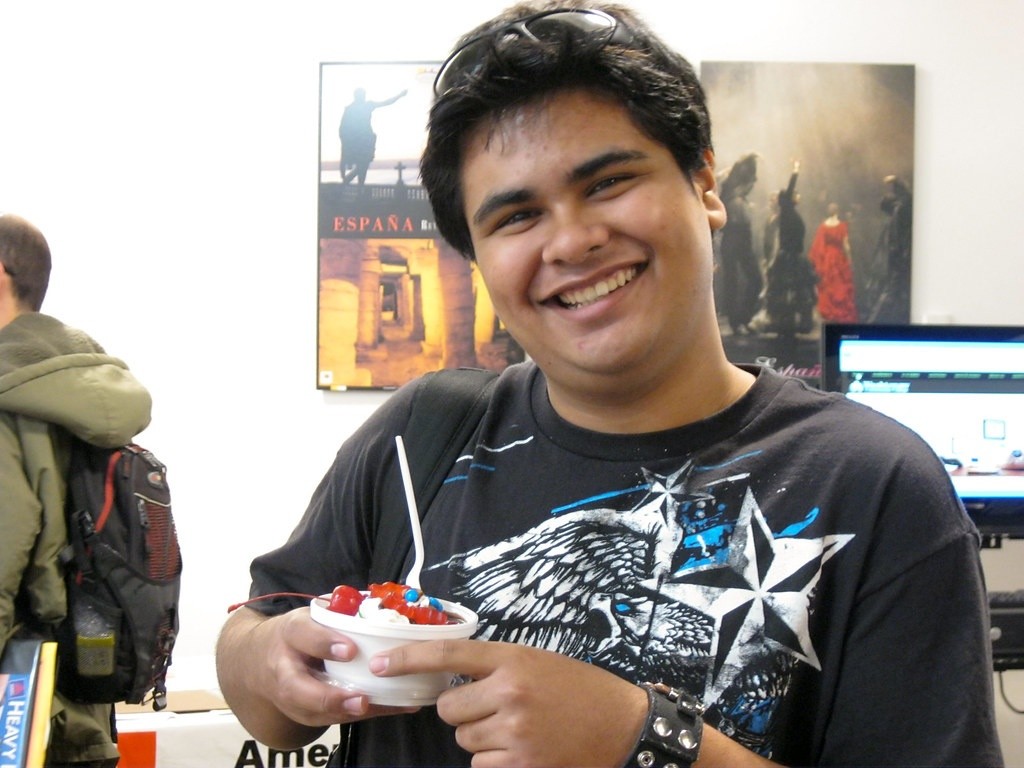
[719,150,914,336]
[0,214,152,767]
[216,0,1005,768]
[338,86,409,185]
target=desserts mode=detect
[326,582,448,625]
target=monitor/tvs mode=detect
[821,323,1024,544]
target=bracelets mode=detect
[619,682,703,768]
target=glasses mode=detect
[432,8,703,107]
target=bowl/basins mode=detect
[313,594,479,708]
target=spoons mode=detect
[395,435,424,594]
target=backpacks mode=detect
[53,438,182,711]
